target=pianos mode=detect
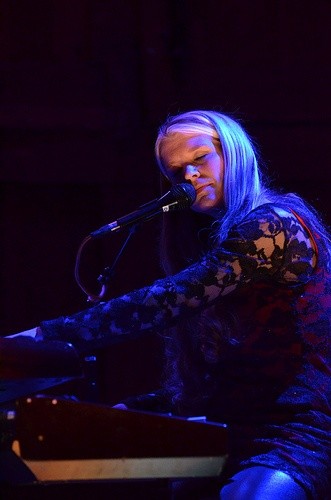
[13,394,231,481]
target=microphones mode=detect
[90,181,196,238]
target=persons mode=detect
[1,108,331,500]
